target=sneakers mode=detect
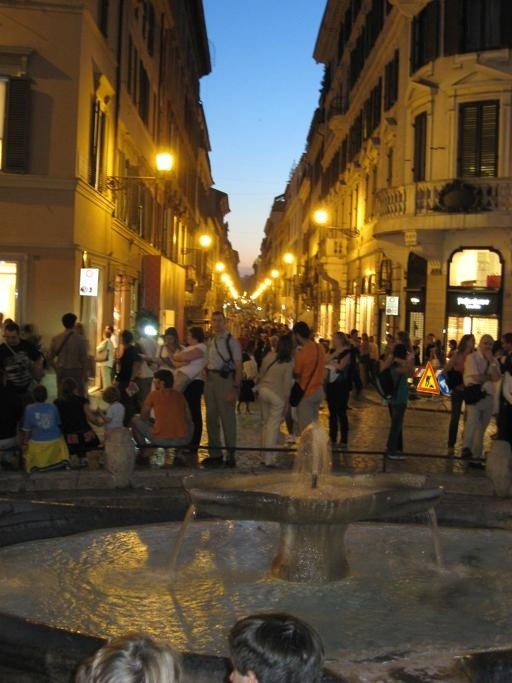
[387,451,407,461]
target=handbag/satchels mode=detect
[95,342,109,363]
[289,343,319,407]
[173,361,205,393]
[464,359,490,404]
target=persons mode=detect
[228,613,324,683]
[200,311,243,466]
[207,305,292,348]
[234,345,255,416]
[324,332,353,448]
[292,321,324,436]
[351,329,512,469]
[0,310,207,473]
[73,631,184,683]
[257,334,296,469]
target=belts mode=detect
[208,369,234,374]
